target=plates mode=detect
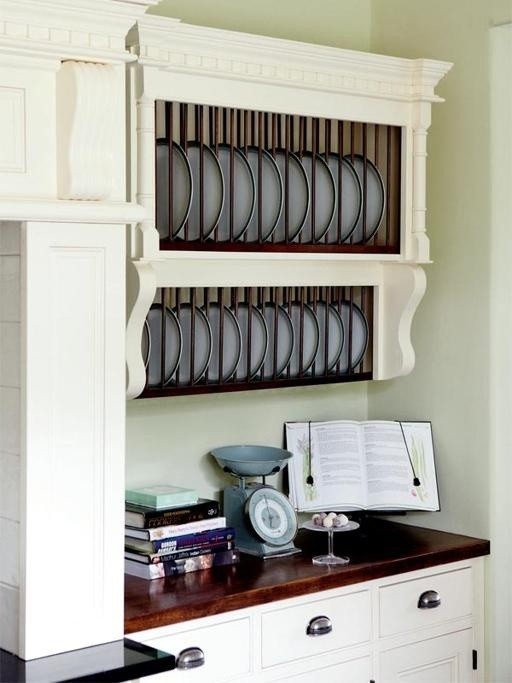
[141,301,371,386]
[156,138,386,243]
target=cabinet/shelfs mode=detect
[376,556,486,683]
[0,222,126,662]
[124,12,454,400]
[256,579,377,680]
[128,607,257,681]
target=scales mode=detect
[211,445,303,560]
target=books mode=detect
[282,420,440,515]
[125,486,240,580]
